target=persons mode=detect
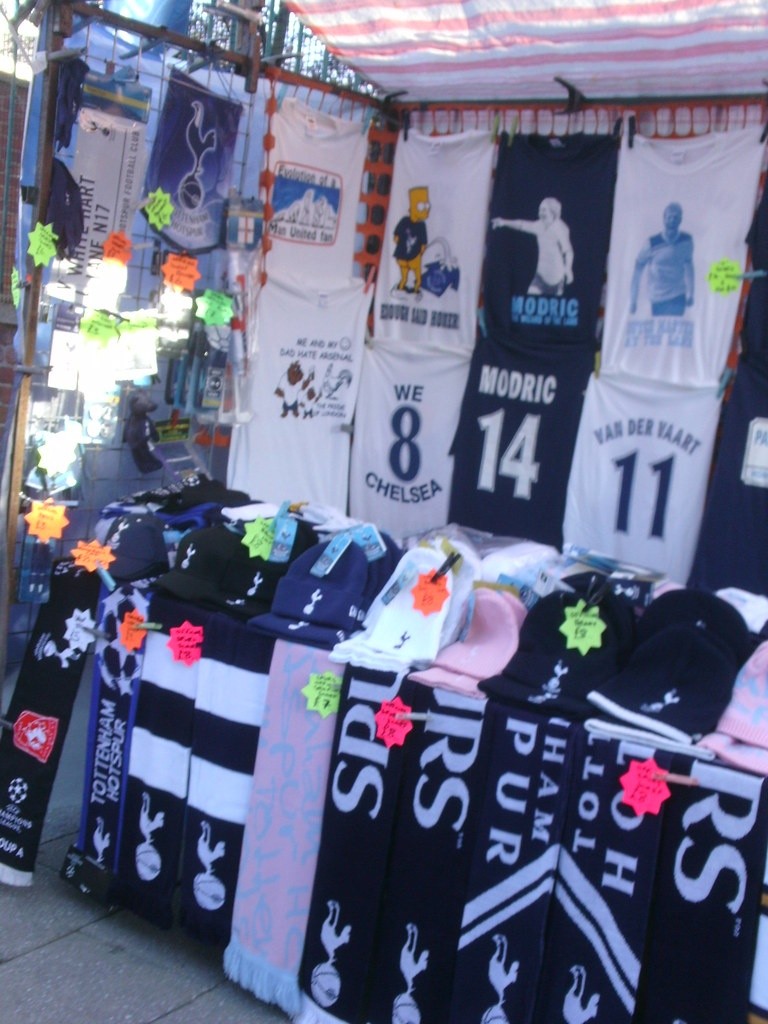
[494,200,575,292]
[630,202,695,315]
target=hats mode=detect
[101,491,768,781]
